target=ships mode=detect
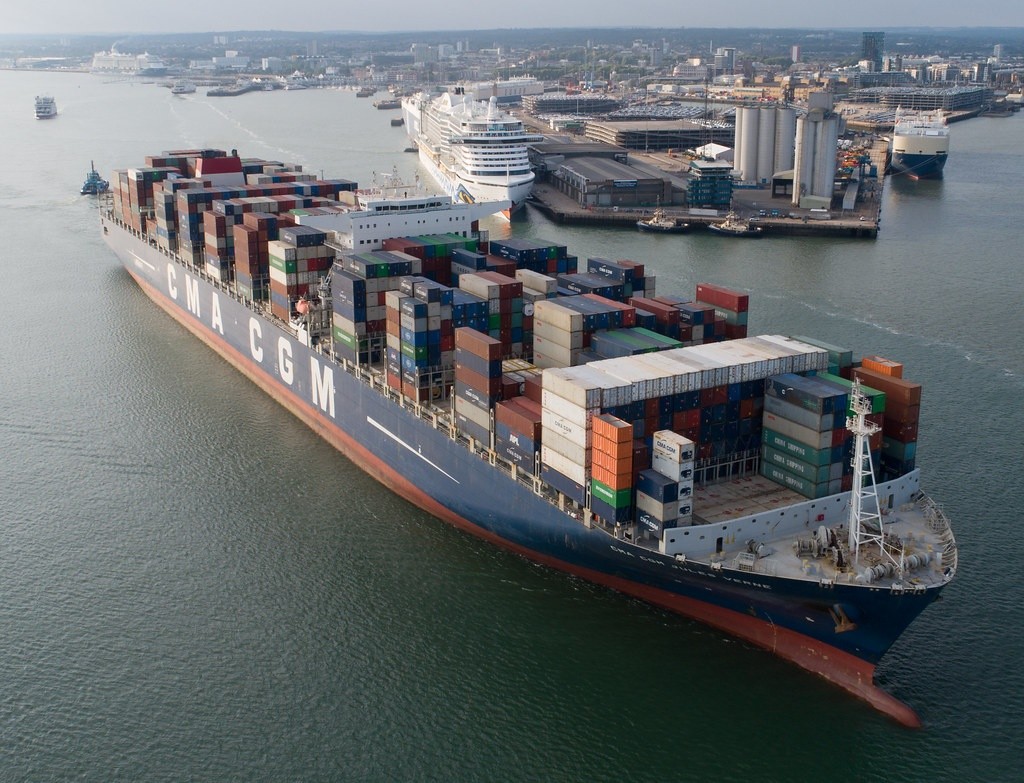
[399,80,536,216]
[97,141,960,729]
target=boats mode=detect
[34,95,58,117]
[706,197,766,237]
[171,76,197,94]
[635,193,691,234]
[80,160,109,196]
[891,102,952,174]
